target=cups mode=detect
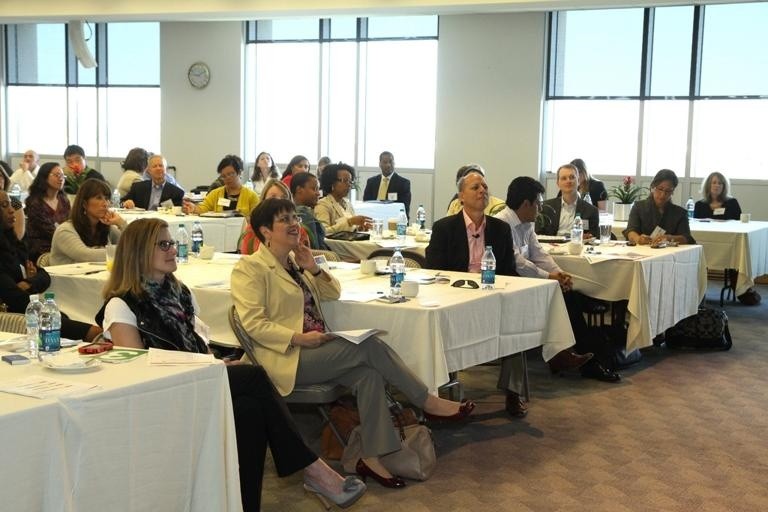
[566,243,583,255]
[599,210,614,242]
[740,214,750,223]
[359,259,375,273]
[388,221,397,230]
[369,218,383,242]
[199,246,215,260]
[401,281,418,296]
[105,245,116,273]
[171,206,182,215]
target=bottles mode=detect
[395,209,407,242]
[685,196,695,224]
[416,204,426,229]
[389,246,405,297]
[25,294,44,357]
[112,189,121,213]
[480,245,497,290]
[38,291,62,361]
[11,181,22,196]
[191,220,203,256]
[569,212,583,245]
[175,224,188,264]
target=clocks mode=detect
[187,62,211,90]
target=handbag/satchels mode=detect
[321,405,436,481]
[664,308,732,350]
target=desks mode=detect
[2,191,767,512]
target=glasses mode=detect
[154,240,176,251]
[275,216,302,224]
[451,280,479,288]
[338,178,354,184]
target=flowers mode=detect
[597,174,653,206]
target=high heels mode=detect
[304,458,405,510]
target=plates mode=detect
[408,272,437,285]
[47,357,101,370]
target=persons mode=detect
[448,158,699,384]
[232,198,475,490]
[695,172,761,305]
[1,145,410,342]
[425,171,595,418]
[95,218,367,512]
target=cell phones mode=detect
[78,342,113,353]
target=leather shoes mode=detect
[506,396,527,417]
[550,350,621,381]
[423,399,476,420]
